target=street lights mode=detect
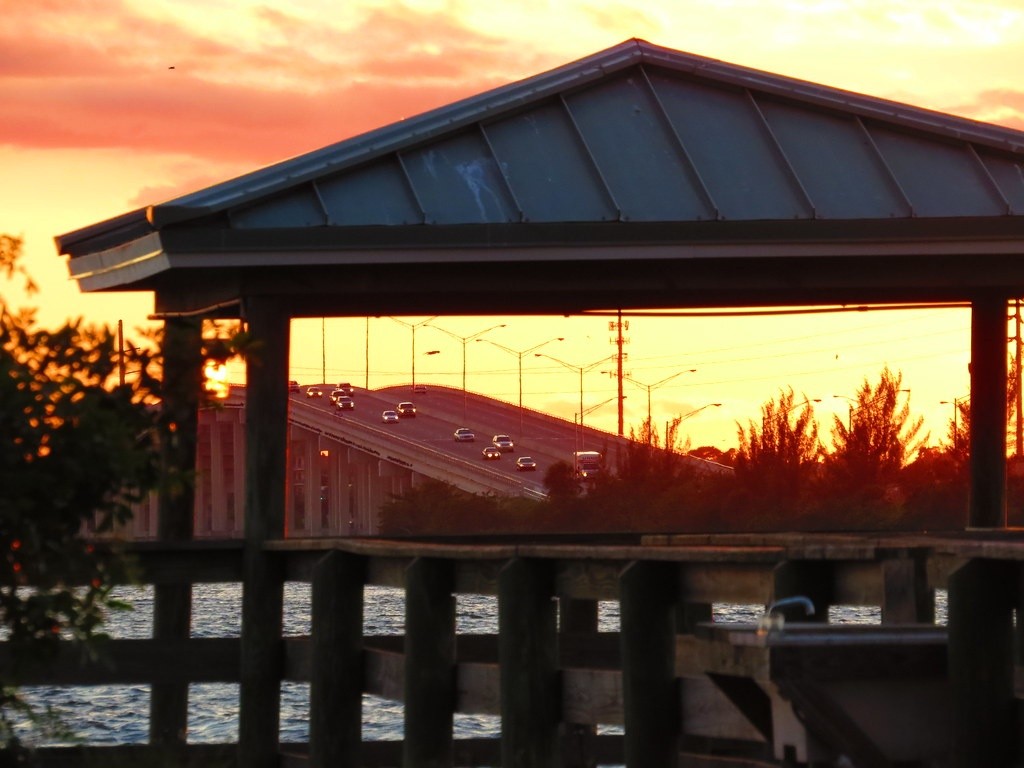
[761,397,822,450]
[600,369,697,447]
[665,402,722,451]
[423,324,507,421]
[534,352,629,450]
[574,395,628,481]
[939,394,971,450]
[375,315,440,404]
[475,336,566,438]
[848,389,910,443]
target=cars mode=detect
[481,446,501,460]
[306,386,322,398]
[329,380,356,410]
[381,409,399,423]
[453,427,475,442]
[288,380,302,393]
[516,456,537,471]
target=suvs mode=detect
[492,435,515,452]
[395,401,418,417]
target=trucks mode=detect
[575,451,603,480]
